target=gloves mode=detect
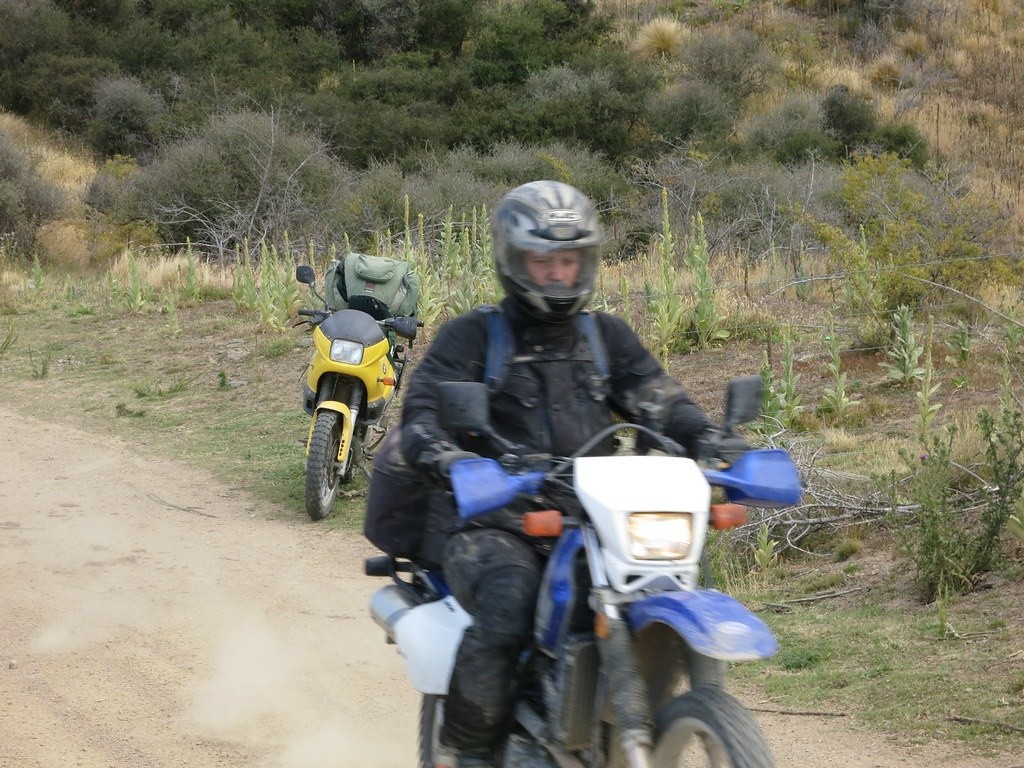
[699,428,753,467]
[437,447,483,479]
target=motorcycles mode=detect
[289,250,422,520]
[362,375,804,768]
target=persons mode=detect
[397,180,750,768]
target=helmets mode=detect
[491,181,604,322]
